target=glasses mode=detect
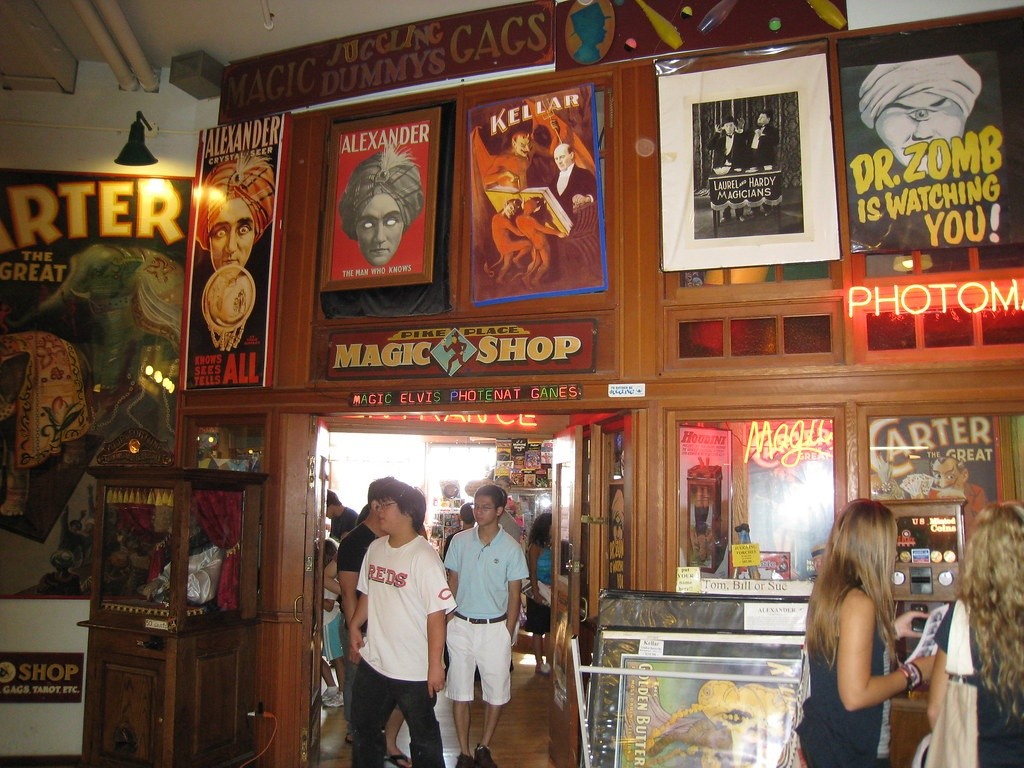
[381,502,397,510]
[470,503,499,510]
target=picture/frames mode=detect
[676,423,732,585]
[321,107,443,292]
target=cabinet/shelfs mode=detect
[77,463,271,767]
[423,436,557,645]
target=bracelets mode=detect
[900,662,922,698]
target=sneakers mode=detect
[455,753,474,768]
[321,688,338,702]
[474,743,497,768]
[324,692,344,707]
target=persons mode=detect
[706,110,778,223]
[796,499,936,768]
[927,505,1024,768]
[316,478,555,768]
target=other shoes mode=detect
[535,663,549,677]
[345,733,353,744]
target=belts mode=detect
[453,611,507,624]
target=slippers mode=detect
[384,753,409,768]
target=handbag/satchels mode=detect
[776,731,808,768]
[922,598,979,768]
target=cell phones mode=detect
[911,603,927,631]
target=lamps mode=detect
[113,111,161,167]
